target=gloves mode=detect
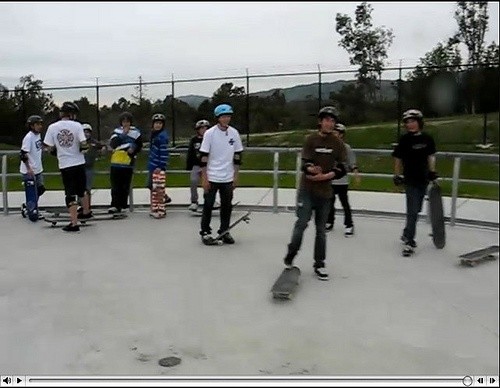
[393,175,404,185]
[429,172,437,180]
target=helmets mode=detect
[214,103,234,117]
[334,123,346,133]
[26,115,43,124]
[402,109,424,123]
[195,120,209,128]
[82,123,93,130]
[151,113,166,120]
[62,102,80,113]
[318,106,339,118]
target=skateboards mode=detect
[43,217,93,228]
[150,168,166,219]
[22,172,38,222]
[271,266,301,301]
[427,180,446,249]
[114,212,129,218]
[46,208,80,216]
[209,211,251,245]
[457,246,500,266]
[189,201,239,212]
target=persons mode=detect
[185,120,219,211]
[19,115,50,222]
[199,105,244,245]
[391,110,438,257]
[148,113,169,216]
[43,101,93,232]
[107,112,143,217]
[325,124,358,236]
[75,123,107,208]
[282,105,348,280]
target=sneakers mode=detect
[315,267,328,280]
[202,234,218,245]
[62,223,80,232]
[212,200,221,207]
[345,227,353,238]
[108,207,117,213]
[38,213,44,220]
[77,206,92,212]
[21,203,27,218]
[403,242,413,256]
[401,235,417,247]
[219,231,235,244]
[77,211,93,219]
[121,208,129,213]
[165,199,171,203]
[189,203,198,210]
[325,223,333,231]
[285,263,293,270]
